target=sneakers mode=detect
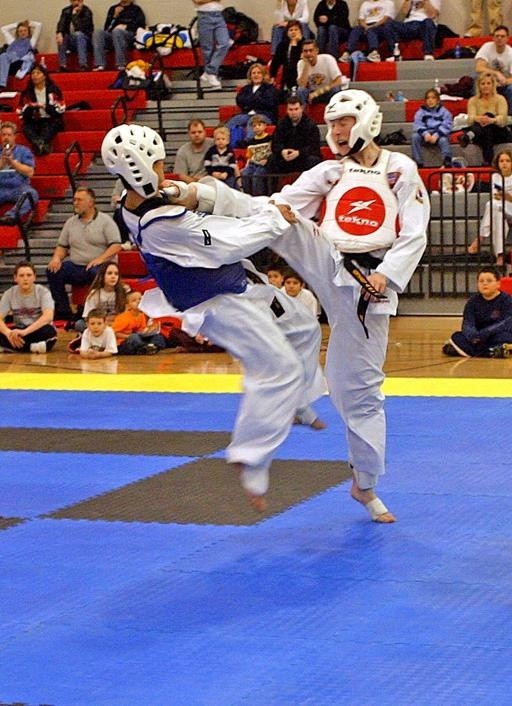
[457,135,469,149]
[55,66,126,73]
[29,341,46,354]
[444,157,451,167]
[32,139,49,155]
[482,161,490,166]
[136,344,158,355]
[385,56,402,62]
[367,50,380,63]
[424,55,434,61]
[338,51,350,62]
[200,71,221,86]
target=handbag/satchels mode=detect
[110,60,173,99]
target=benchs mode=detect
[0,35,511,306]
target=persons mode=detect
[466,147,512,266]
[100,122,331,511]
[0,1,147,224]
[173,0,442,195]
[443,266,511,360]
[265,263,318,317]
[411,0,512,167]
[159,87,434,524]
[0,185,165,356]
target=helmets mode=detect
[323,89,382,154]
[100,124,165,198]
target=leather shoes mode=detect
[53,312,73,320]
[0,216,14,225]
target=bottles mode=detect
[236,83,241,94]
[455,41,461,59]
[392,43,401,59]
[39,57,47,70]
[291,88,297,96]
[434,77,441,93]
